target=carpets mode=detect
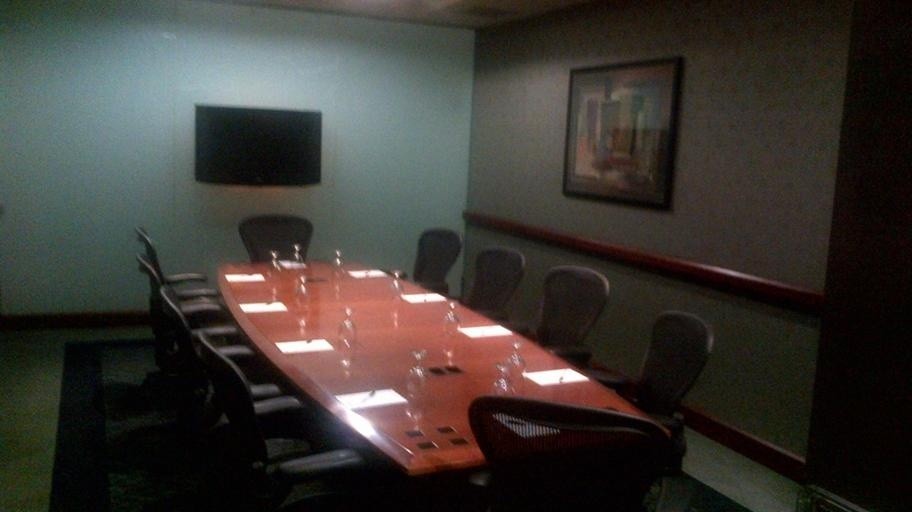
[49,339,750,512]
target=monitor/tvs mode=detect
[193,105,322,187]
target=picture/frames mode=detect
[562,57,681,210]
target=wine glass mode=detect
[405,348,429,393]
[266,250,282,277]
[329,248,346,278]
[508,340,527,380]
[289,243,307,274]
[440,301,463,339]
[388,267,404,301]
[292,276,310,313]
[492,361,515,397]
[338,305,357,341]
